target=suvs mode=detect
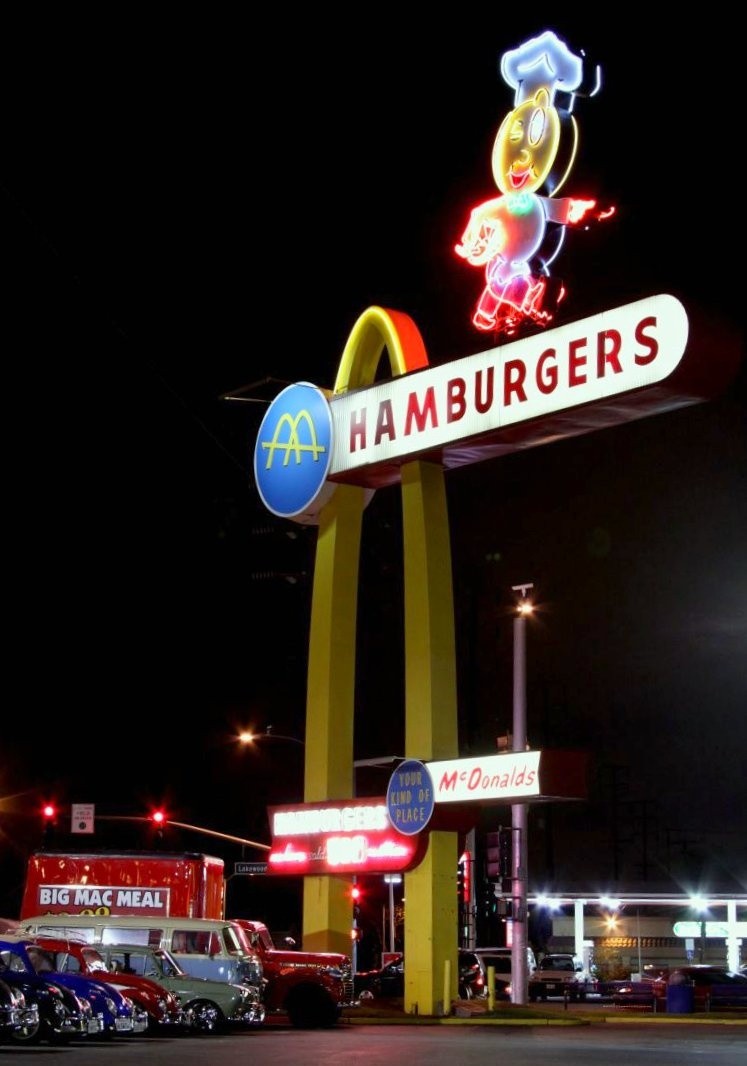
[352,946,488,1007]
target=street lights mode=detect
[690,894,707,963]
[509,583,536,1005]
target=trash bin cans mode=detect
[667,983,696,1014]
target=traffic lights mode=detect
[149,799,167,850]
[492,898,506,916]
[352,883,362,918]
[486,825,505,879]
[456,850,470,902]
[38,790,57,831]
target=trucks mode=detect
[19,850,352,1030]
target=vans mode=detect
[469,947,542,999]
[4,911,270,990]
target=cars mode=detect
[612,962,747,1012]
[57,943,266,1039]
[0,933,187,1048]
[527,953,587,1003]
